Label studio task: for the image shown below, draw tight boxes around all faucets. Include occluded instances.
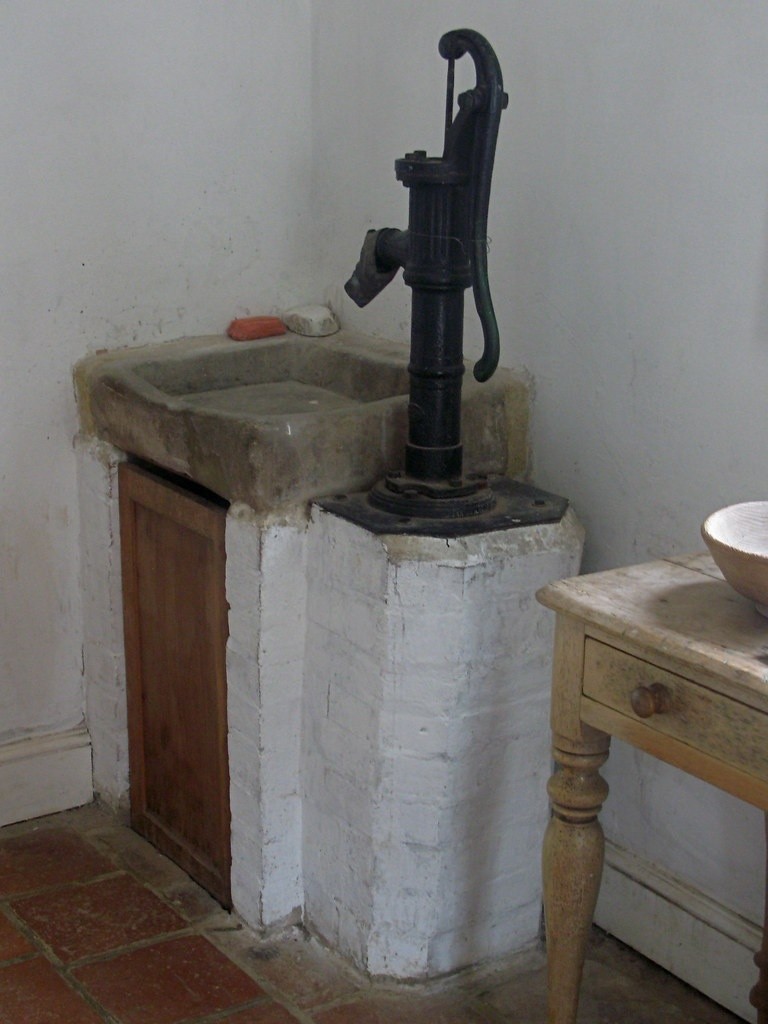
[343,227,404,310]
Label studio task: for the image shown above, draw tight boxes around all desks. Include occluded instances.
[535,550,768,1024]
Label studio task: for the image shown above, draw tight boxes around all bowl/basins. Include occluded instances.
[700,501,768,618]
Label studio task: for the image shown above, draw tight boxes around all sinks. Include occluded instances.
[73,327,532,514]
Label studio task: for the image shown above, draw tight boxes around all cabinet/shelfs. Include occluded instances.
[116,457,232,913]
